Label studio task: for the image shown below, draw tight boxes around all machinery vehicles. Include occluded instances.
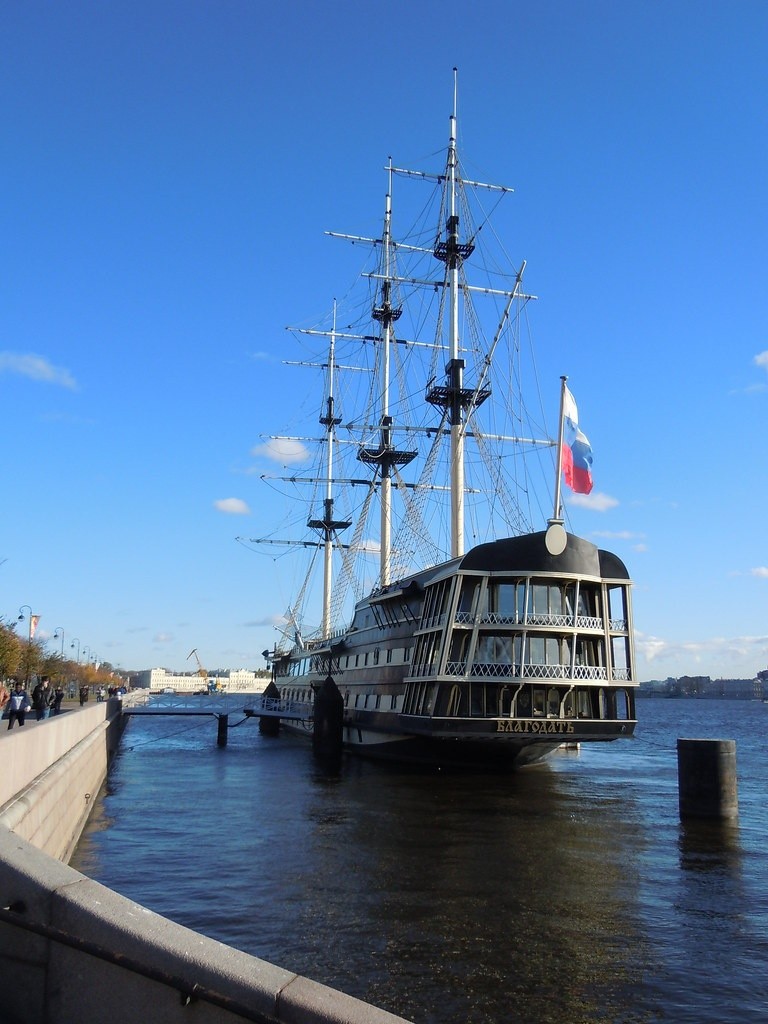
[186,648,227,696]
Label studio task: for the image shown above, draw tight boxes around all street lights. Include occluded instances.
[82,646,105,666]
[53,626,65,661]
[71,637,80,663]
[18,605,33,644]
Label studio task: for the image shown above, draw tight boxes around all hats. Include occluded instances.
[40,676,50,681]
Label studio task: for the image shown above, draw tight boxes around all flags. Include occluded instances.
[559,386,592,494]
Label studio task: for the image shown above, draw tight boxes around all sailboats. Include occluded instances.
[249,67,640,775]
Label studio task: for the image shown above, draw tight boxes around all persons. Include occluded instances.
[0,678,9,722]
[31,676,55,722]
[55,685,128,714]
[6,682,32,731]
